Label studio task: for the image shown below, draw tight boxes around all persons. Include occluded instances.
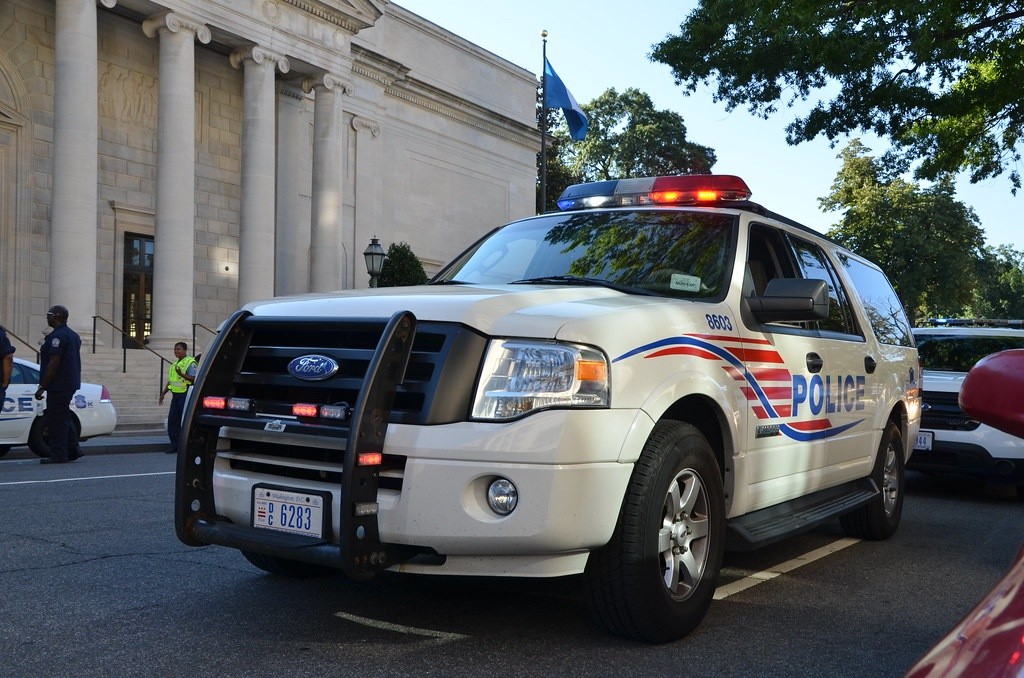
[35,305,85,464]
[159,342,199,453]
[0,325,16,417]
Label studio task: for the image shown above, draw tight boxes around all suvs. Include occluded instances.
[165,174,928,642]
[907,319,1024,486]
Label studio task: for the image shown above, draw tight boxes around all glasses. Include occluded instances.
[47,311,59,317]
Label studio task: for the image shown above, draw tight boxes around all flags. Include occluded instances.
[544,59,588,140]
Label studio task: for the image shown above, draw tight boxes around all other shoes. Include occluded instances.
[41,454,68,464]
[67,447,85,461]
[165,444,177,453]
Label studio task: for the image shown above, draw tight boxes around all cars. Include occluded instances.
[0,354,118,457]
[897,349,1023,678]
